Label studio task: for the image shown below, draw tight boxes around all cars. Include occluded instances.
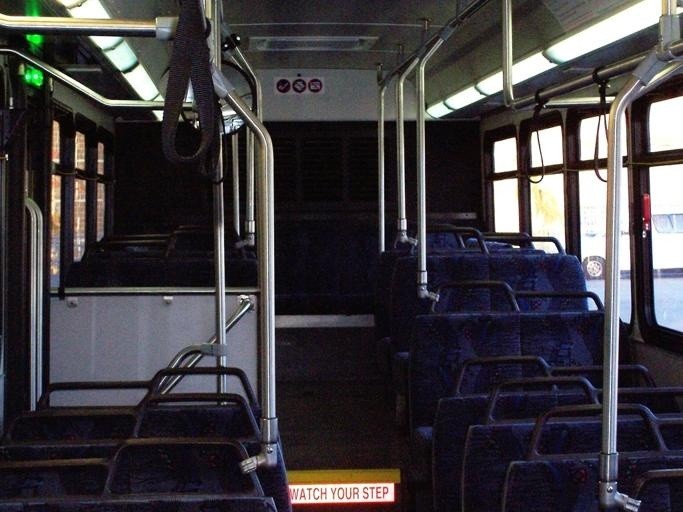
[581,212,683,279]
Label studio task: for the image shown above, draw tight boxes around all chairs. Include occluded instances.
[2,224,683,512]
